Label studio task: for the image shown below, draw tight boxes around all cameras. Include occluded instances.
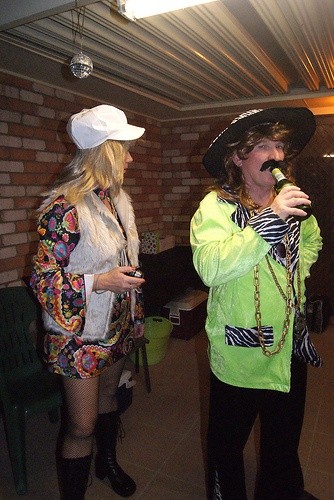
[123,266,145,278]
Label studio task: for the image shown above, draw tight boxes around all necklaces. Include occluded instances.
[243,186,300,357]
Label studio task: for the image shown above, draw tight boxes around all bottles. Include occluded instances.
[272,167,312,221]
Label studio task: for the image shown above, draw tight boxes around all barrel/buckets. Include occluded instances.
[128,317,173,365]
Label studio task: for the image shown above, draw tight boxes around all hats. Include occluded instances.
[66,104,146,150]
[202,105,317,179]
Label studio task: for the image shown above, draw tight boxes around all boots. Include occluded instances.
[56,454,92,500]
[95,410,137,497]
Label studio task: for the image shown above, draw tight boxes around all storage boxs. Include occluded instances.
[129,317,173,365]
[162,286,209,341]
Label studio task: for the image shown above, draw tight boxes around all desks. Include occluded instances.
[136,246,196,308]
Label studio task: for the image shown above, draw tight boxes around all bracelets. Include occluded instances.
[95,273,108,294]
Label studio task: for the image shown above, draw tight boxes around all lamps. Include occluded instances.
[70,0,94,80]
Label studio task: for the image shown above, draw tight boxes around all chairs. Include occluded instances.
[0,286,67,495]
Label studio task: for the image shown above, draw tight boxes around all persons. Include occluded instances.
[190,106,323,500]
[29,105,145,500]
